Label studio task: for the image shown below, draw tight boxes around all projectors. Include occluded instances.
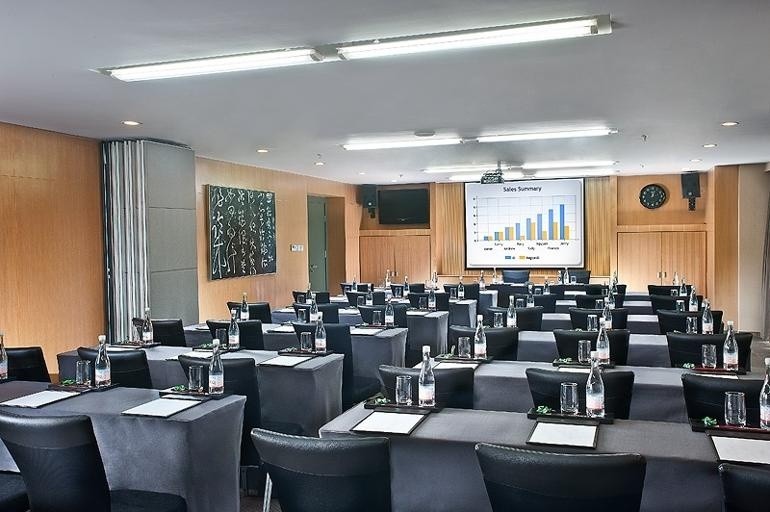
[479,169,505,185]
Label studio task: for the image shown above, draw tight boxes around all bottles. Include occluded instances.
[215,267,499,352]
[597,321,610,365]
[586,271,714,334]
[473,314,487,359]
[492,268,577,329]
[208,339,224,394]
[760,358,770,430]
[419,345,436,405]
[584,351,607,418]
[724,320,739,371]
[94,333,110,387]
[0,333,8,383]
[141,307,154,343]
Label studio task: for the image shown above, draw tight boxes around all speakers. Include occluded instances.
[360,183,377,208]
[680,174,701,199]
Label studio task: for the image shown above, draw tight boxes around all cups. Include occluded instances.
[700,344,717,369]
[395,375,413,405]
[458,335,471,359]
[130,325,141,342]
[187,364,204,391]
[579,340,591,365]
[560,380,579,415]
[75,359,90,384]
[724,390,746,428]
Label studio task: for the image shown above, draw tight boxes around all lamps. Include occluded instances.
[341,118,616,185]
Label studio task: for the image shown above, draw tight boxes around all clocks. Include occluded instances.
[639,182,667,210]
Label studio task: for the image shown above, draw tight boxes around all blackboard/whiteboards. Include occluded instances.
[204,183,276,281]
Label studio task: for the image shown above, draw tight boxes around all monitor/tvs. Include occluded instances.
[378,187,430,225]
